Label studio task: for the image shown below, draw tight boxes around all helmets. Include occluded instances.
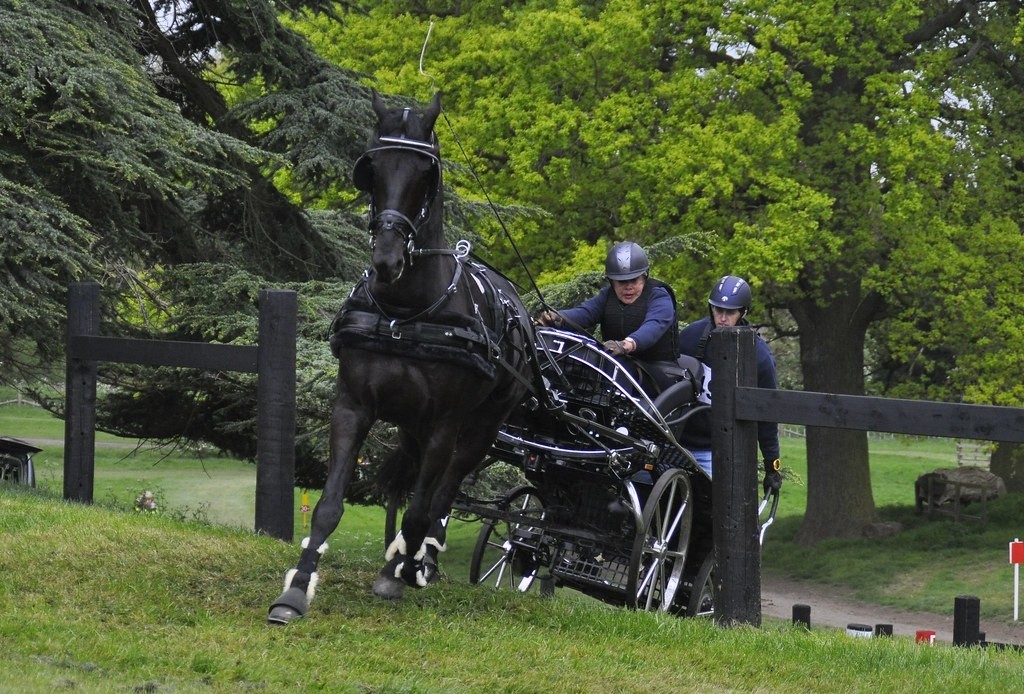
[605,242,649,281]
[708,274,751,309]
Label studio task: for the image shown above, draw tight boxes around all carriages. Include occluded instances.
[267,87,781,624]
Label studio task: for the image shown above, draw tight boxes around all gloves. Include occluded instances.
[763,458,782,497]
[603,339,627,357]
[539,308,563,329]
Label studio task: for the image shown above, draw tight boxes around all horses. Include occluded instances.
[269,87,535,627]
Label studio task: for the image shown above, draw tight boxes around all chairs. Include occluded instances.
[634,351,704,441]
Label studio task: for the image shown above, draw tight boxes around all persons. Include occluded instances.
[679,276,784,494]
[533,240,682,577]
